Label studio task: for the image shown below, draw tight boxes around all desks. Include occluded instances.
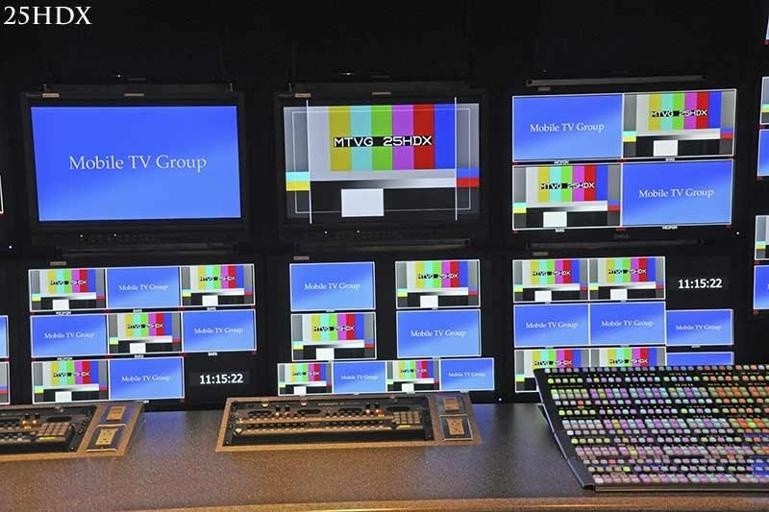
[0,402,769,512]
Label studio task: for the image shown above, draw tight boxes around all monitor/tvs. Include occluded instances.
[0,77,769,412]
